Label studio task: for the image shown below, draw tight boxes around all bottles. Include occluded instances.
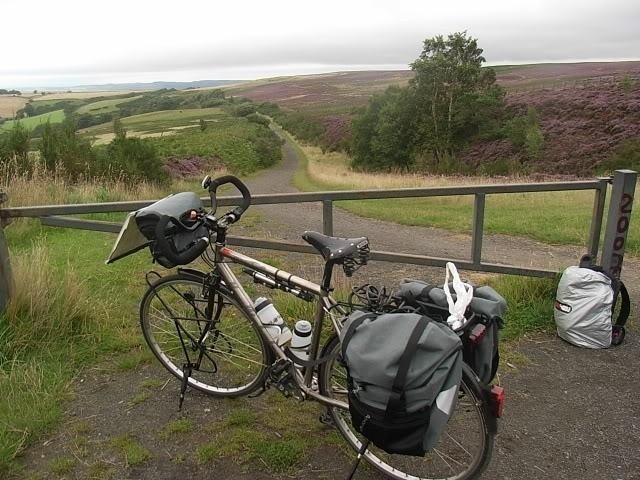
[252,295,313,370]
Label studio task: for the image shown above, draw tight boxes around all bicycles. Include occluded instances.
[140,174,508,480]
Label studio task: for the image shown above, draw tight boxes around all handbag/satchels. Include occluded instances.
[554,254,630,350]
[338,310,465,457]
[398,277,507,383]
